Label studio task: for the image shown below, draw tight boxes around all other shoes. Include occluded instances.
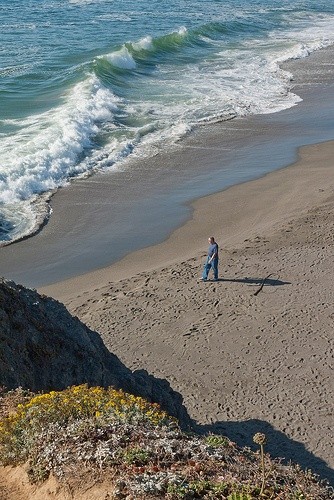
[199,277,207,282]
[211,277,218,281]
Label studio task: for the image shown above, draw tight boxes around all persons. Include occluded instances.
[199,237,219,282]
